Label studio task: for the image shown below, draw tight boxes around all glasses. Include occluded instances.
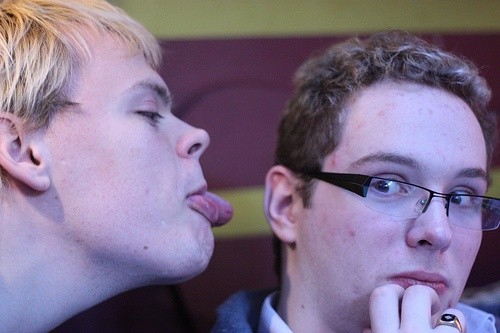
[299,159,500,231]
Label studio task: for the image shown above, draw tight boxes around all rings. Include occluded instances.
[434,313,463,333]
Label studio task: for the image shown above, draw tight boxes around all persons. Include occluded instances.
[209,27,500,333]
[0,0,234,333]
